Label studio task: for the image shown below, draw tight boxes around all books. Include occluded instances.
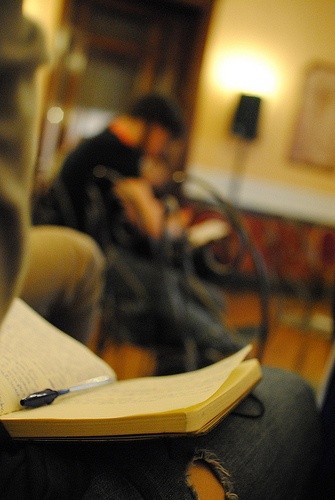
[1,295,263,442]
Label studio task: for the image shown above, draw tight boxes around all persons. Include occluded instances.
[1,365,319,500]
[39,95,244,363]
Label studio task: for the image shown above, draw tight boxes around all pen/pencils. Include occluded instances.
[19,376,113,408]
